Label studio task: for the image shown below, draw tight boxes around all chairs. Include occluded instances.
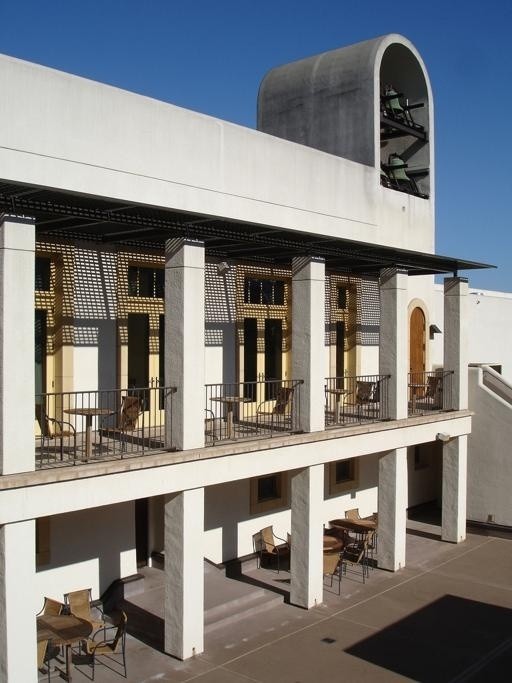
[342,380,376,424]
[34,403,77,461]
[256,507,377,596]
[85,611,129,681]
[63,588,108,642]
[36,596,66,618]
[254,387,295,437]
[204,408,218,442]
[425,376,443,411]
[97,395,146,457]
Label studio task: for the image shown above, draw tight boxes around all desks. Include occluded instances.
[408,384,424,413]
[37,615,93,683]
[210,397,253,439]
[61,408,115,457]
[325,389,351,423]
